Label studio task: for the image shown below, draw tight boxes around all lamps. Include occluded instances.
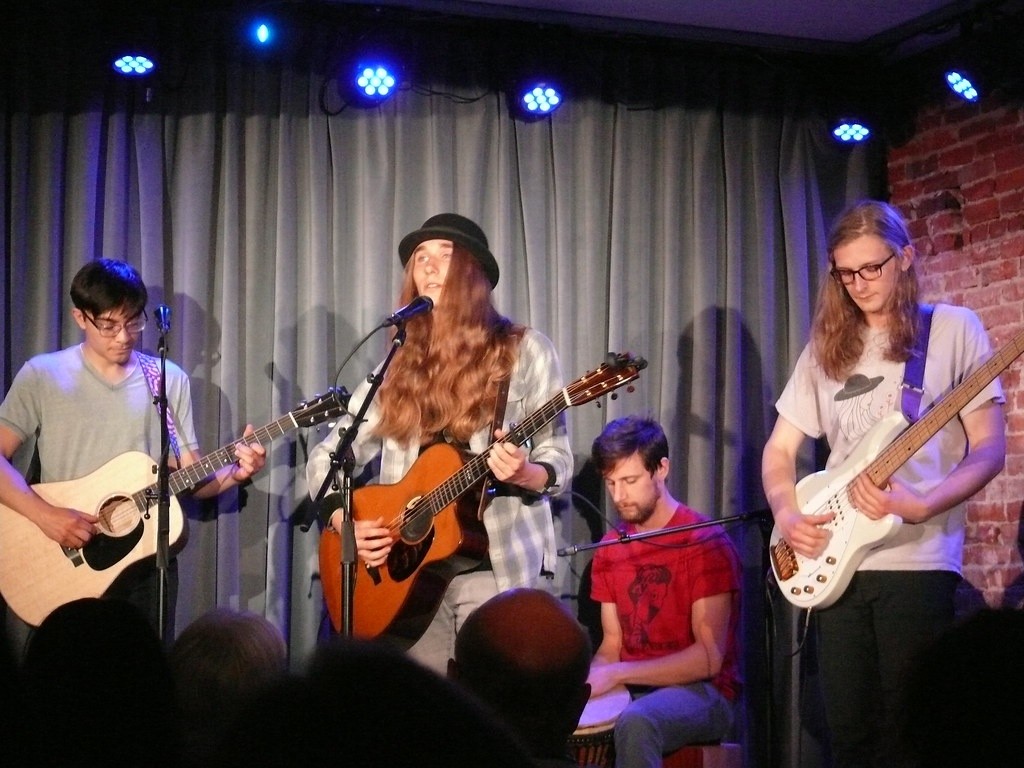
[338,27,406,110]
[942,26,1016,103]
[108,4,162,82]
[826,69,879,144]
[491,38,575,123]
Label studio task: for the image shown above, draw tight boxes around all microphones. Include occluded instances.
[382,296,433,327]
[154,304,170,334]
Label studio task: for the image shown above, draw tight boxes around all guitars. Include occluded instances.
[0,382,351,635]
[766,325,1023,613]
[314,347,650,653]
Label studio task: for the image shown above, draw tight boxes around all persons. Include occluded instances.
[305,214,572,672]
[760,203,1004,768]
[577,417,742,768]
[1,588,593,768]
[0,258,269,636]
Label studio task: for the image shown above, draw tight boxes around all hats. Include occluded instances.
[398,213,500,290]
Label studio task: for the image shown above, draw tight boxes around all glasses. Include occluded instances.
[83,309,149,338]
[831,254,894,285]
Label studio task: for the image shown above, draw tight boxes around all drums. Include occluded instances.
[563,666,633,767]
